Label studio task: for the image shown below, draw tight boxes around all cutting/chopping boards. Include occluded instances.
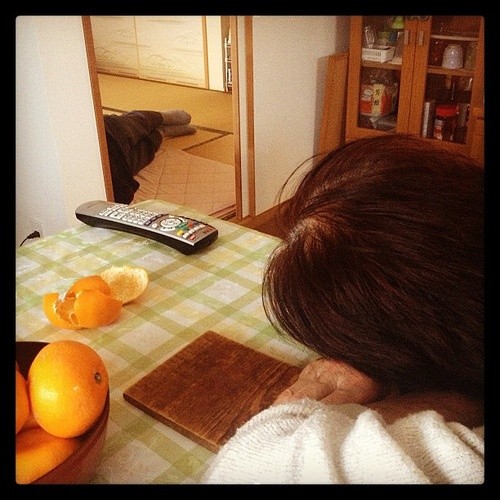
[123,329,302,453]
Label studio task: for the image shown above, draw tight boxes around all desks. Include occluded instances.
[15,198,325,484]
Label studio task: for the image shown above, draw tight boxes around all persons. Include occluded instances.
[104,111,197,205]
[263,142,485,428]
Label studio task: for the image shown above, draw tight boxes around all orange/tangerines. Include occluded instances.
[15,339,109,485]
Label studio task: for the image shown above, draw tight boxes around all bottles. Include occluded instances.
[428,40,442,66]
[433,75,474,144]
[359,68,399,117]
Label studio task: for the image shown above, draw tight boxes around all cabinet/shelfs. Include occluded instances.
[346,17,484,163]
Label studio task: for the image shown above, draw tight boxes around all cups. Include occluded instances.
[377,28,396,46]
[442,43,463,70]
[464,41,477,71]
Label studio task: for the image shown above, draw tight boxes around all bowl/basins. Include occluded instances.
[16,340,110,484]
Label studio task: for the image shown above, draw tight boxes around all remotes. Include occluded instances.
[75,200,218,254]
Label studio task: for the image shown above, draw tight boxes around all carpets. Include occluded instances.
[129,147,237,214]
